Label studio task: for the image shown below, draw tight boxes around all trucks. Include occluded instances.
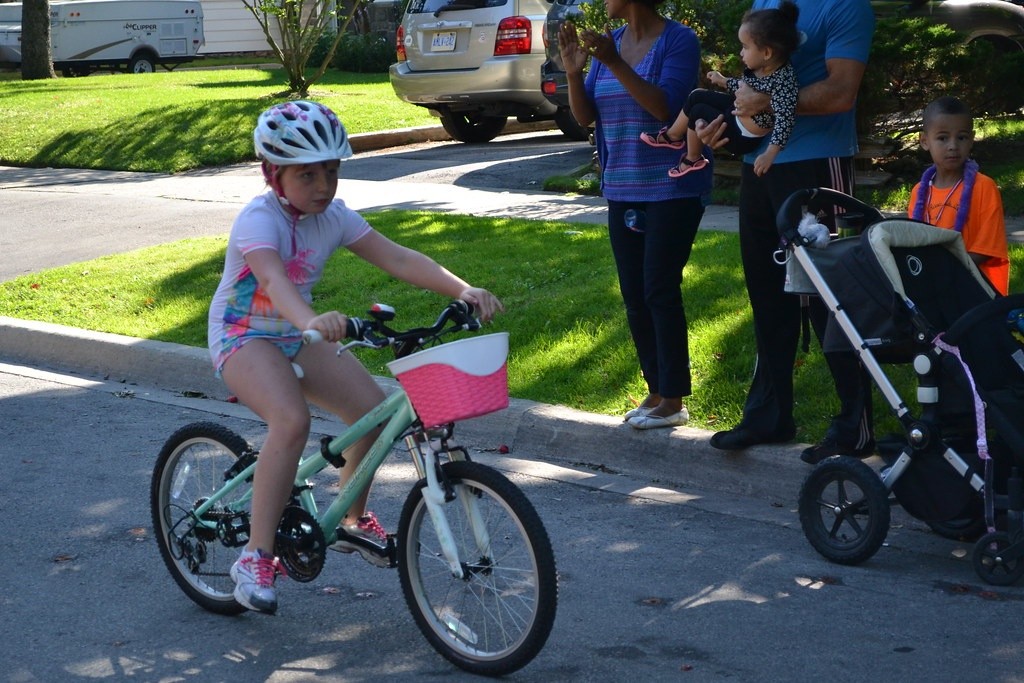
[2,0,205,76]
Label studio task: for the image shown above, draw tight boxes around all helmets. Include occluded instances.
[255,101,354,164]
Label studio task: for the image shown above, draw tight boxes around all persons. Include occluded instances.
[905,95,1011,303]
[694,0,878,464]
[207,99,505,615]
[557,0,715,430]
[638,7,800,178]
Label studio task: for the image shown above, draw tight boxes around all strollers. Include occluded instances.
[772,188,1023,586]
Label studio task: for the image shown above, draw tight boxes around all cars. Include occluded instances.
[390,0,591,141]
[540,0,1023,111]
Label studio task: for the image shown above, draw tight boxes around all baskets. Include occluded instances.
[388,331,512,428]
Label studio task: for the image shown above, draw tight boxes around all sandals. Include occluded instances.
[669,154,710,177]
[639,132,686,149]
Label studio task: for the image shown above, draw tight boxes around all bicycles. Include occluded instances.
[151,299,560,677]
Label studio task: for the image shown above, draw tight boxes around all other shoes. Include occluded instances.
[625,406,690,427]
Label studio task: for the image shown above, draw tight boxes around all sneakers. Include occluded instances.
[331,514,399,568]
[799,432,879,462]
[710,426,798,449]
[231,543,279,613]
